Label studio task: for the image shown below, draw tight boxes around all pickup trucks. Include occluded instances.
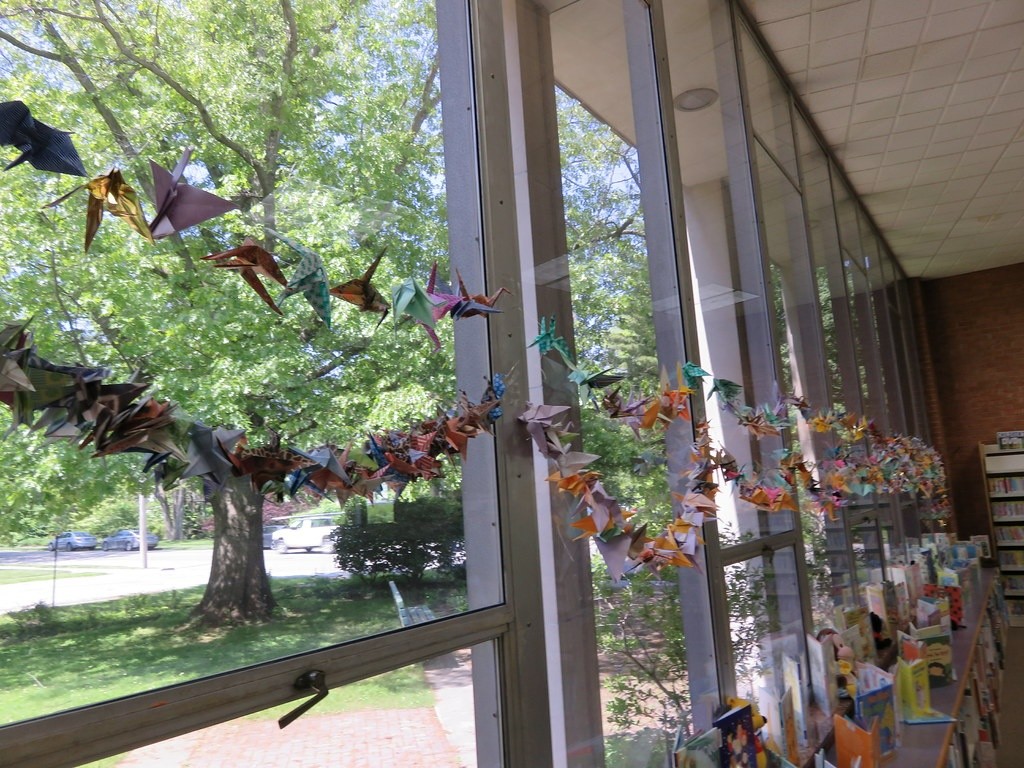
[271,517,341,554]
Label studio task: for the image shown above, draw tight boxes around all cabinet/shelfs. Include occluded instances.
[806,442,1024,768]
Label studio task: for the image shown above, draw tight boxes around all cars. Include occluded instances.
[48,531,98,551]
[102,528,159,552]
[263,516,294,550]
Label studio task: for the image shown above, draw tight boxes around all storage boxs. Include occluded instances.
[997,430,1024,451]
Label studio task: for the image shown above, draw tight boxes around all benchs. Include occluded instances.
[389,580,437,628]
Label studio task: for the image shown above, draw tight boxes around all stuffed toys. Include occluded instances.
[816,628,858,698]
[727,698,768,768]
[869,611,893,650]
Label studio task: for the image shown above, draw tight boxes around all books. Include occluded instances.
[815,749,825,768]
[989,476,1024,617]
[855,534,1008,768]
[833,715,881,768]
[671,704,758,768]
[759,687,799,765]
[806,634,839,716]
[782,652,820,748]
[824,755,862,768]
[997,431,1024,450]
